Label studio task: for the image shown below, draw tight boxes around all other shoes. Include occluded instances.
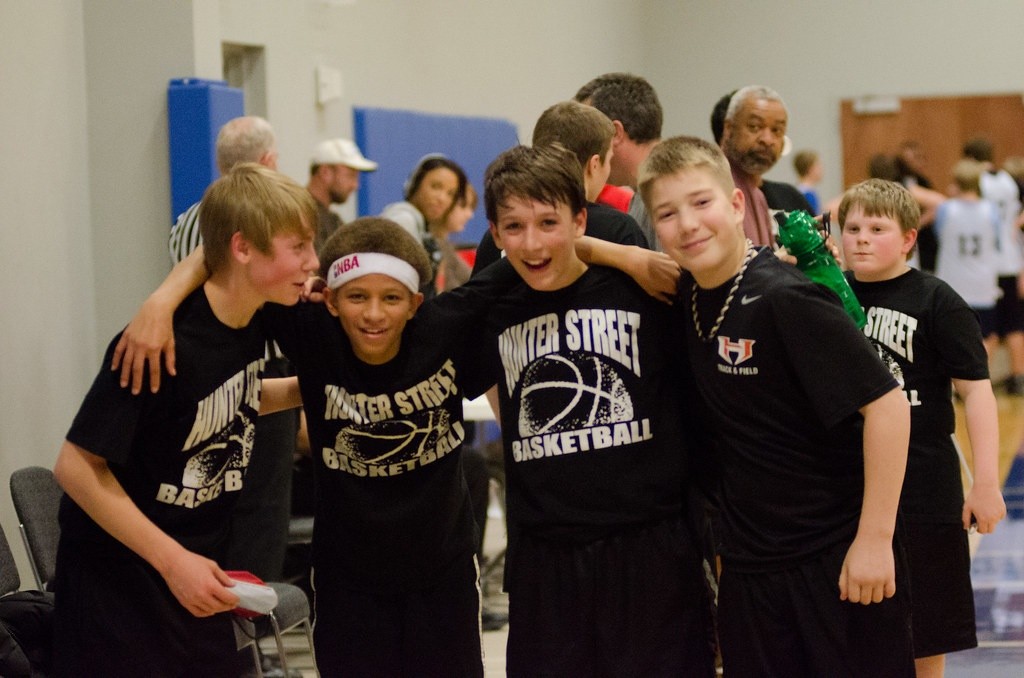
[1006,372,1023,397]
[481,604,509,631]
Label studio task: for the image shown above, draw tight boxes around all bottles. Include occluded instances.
[773,209,867,328]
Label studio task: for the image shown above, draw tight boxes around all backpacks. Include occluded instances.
[0,590,56,678]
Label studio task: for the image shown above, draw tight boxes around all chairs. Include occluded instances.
[0,462,315,678]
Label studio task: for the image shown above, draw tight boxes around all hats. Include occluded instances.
[310,138,379,172]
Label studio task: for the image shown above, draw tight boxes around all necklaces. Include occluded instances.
[692,239,752,345]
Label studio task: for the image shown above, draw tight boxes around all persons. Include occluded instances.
[111,218,681,678]
[52,161,320,678]
[638,135,916,678]
[839,178,1005,678]
[169,76,1024,633]
[299,146,717,678]
